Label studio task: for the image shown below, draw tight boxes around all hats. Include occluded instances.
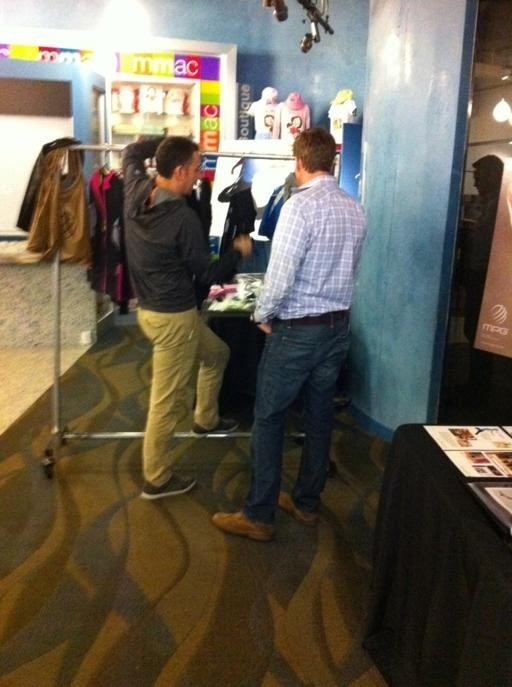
[335,89,353,103]
[287,92,303,109]
[262,87,277,99]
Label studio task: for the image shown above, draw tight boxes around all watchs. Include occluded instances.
[249,314,262,326]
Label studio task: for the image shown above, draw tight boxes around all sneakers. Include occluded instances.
[141,472,197,498]
[192,418,240,436]
[278,491,319,525]
[211,510,271,541]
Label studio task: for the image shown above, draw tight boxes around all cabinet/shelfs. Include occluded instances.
[104,73,202,173]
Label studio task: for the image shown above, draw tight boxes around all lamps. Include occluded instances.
[261,0,321,54]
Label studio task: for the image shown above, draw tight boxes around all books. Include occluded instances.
[466,481,511,531]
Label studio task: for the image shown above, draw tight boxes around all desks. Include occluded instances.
[353,411,512,687]
[202,287,268,435]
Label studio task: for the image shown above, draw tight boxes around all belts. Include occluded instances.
[290,311,343,324]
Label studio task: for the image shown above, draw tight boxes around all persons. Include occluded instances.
[210,127,366,542]
[286,116,302,138]
[458,154,503,421]
[263,115,274,132]
[121,135,253,501]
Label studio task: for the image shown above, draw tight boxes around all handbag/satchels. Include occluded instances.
[16,137,93,264]
